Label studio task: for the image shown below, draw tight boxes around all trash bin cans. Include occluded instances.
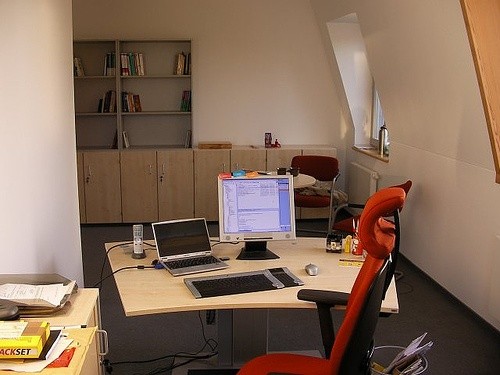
[367,345,429,375]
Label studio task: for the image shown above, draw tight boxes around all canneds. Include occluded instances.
[265,133,272,148]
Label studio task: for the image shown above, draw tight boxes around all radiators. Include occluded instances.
[348,161,379,204]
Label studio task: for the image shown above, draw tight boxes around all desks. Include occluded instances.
[80,327,108,375]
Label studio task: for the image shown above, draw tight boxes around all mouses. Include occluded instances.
[305,263,319,276]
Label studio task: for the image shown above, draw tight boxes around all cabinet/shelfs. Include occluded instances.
[121,150,194,224]
[78,151,120,224]
[74,37,120,148]
[87,295,101,326]
[195,150,267,222]
[119,37,196,149]
[104,236,400,374]
[267,149,336,220]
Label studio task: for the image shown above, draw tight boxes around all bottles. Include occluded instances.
[379,122,387,154]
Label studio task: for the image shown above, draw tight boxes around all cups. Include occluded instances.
[290,166,300,177]
[276,167,286,175]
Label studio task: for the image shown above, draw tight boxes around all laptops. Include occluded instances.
[151,218,229,277]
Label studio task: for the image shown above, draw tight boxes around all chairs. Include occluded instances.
[289,155,341,237]
[235,180,412,375]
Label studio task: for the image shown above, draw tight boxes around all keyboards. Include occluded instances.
[184,267,305,299]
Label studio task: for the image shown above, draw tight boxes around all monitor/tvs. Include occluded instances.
[218,175,296,260]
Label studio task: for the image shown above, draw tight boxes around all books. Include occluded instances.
[185,129,191,148]
[96,91,116,112]
[122,131,130,148]
[111,129,116,148]
[174,52,190,75]
[120,53,145,75]
[74,56,85,76]
[39,330,62,360]
[122,92,142,112]
[180,91,190,112]
[103,52,115,76]
[197,143,232,149]
